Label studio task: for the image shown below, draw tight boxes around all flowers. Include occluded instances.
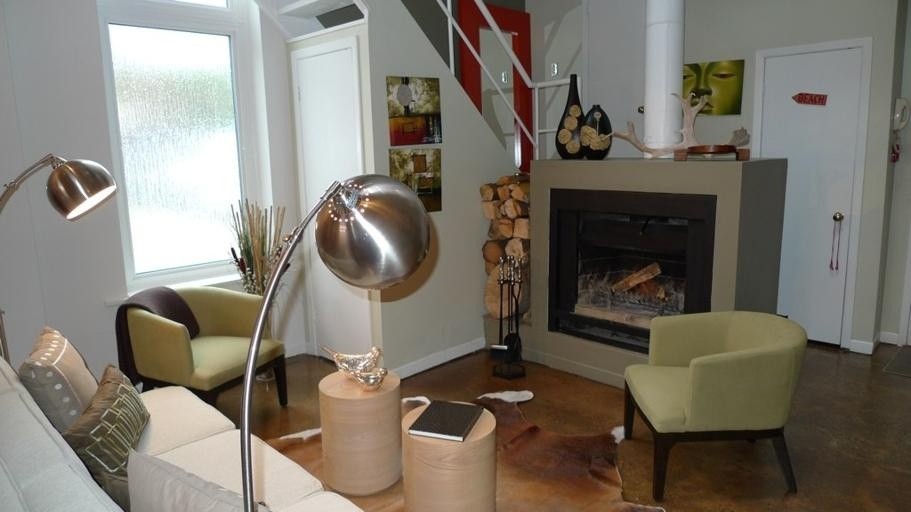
[226,197,291,382]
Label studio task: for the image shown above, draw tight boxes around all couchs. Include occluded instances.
[1,355,364,511]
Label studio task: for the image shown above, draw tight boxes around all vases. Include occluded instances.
[555,72,613,161]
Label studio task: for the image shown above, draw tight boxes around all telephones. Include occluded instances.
[893,98,910,130]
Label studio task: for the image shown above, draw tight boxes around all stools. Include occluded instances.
[318,368,496,511]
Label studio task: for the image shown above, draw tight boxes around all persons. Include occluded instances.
[684,61,743,116]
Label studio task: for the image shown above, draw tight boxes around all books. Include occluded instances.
[408,400,483,442]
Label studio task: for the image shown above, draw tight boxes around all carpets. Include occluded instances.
[264,389,665,510]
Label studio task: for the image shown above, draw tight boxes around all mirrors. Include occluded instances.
[476,24,524,169]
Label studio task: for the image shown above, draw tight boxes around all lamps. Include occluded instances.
[1,152,119,222]
[236,172,437,512]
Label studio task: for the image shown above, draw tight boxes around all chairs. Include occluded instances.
[623,309,809,502]
[125,284,287,407]
